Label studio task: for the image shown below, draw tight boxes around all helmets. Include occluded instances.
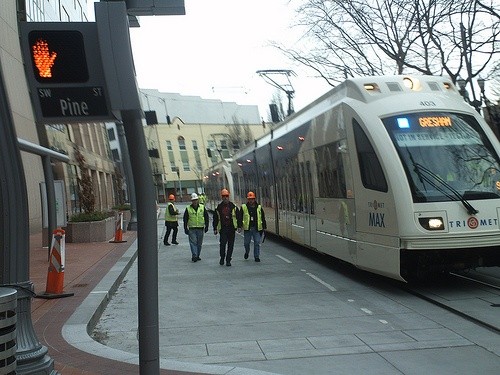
[247,192,255,198]
[190,192,198,200]
[221,189,229,195]
[169,195,175,199]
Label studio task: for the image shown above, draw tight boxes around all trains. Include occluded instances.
[201,75,500,284]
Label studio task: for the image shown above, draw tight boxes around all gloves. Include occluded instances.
[204,227,208,233]
[214,230,218,235]
[237,228,241,233]
[185,229,189,234]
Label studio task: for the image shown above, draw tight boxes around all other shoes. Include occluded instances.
[172,241,179,244]
[226,260,231,266]
[192,257,197,262]
[244,252,249,259]
[164,242,171,246]
[220,257,225,265]
[255,258,260,261]
[197,255,202,260]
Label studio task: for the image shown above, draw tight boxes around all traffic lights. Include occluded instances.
[18,21,110,124]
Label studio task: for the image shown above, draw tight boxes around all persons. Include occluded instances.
[213,188,243,266]
[164,195,179,246]
[239,191,266,262]
[183,193,209,261]
[430,154,459,192]
